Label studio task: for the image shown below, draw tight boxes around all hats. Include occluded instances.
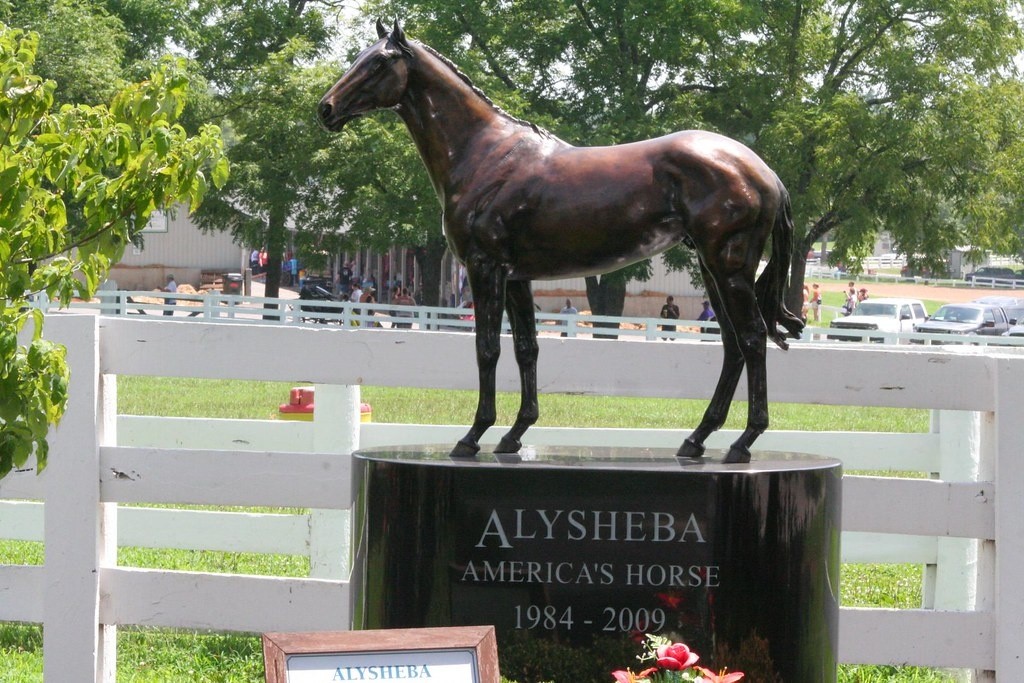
[701,301,709,307]
[363,286,376,292]
[859,288,867,294]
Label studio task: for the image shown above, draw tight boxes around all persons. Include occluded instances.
[560,298,577,337]
[342,283,377,327]
[697,301,715,334]
[156,274,177,315]
[660,296,679,341]
[801,283,822,322]
[842,282,857,315]
[251,246,306,290]
[389,285,417,328]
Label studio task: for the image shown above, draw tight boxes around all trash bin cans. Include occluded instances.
[221,272,243,306]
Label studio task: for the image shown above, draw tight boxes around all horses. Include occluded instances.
[315,15,809,466]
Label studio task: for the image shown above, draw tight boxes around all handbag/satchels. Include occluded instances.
[841,306,848,315]
[817,300,821,304]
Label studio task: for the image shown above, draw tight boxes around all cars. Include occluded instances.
[964,265,1014,286]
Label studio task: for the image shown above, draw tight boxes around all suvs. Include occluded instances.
[908,297,1024,348]
[822,298,927,345]
